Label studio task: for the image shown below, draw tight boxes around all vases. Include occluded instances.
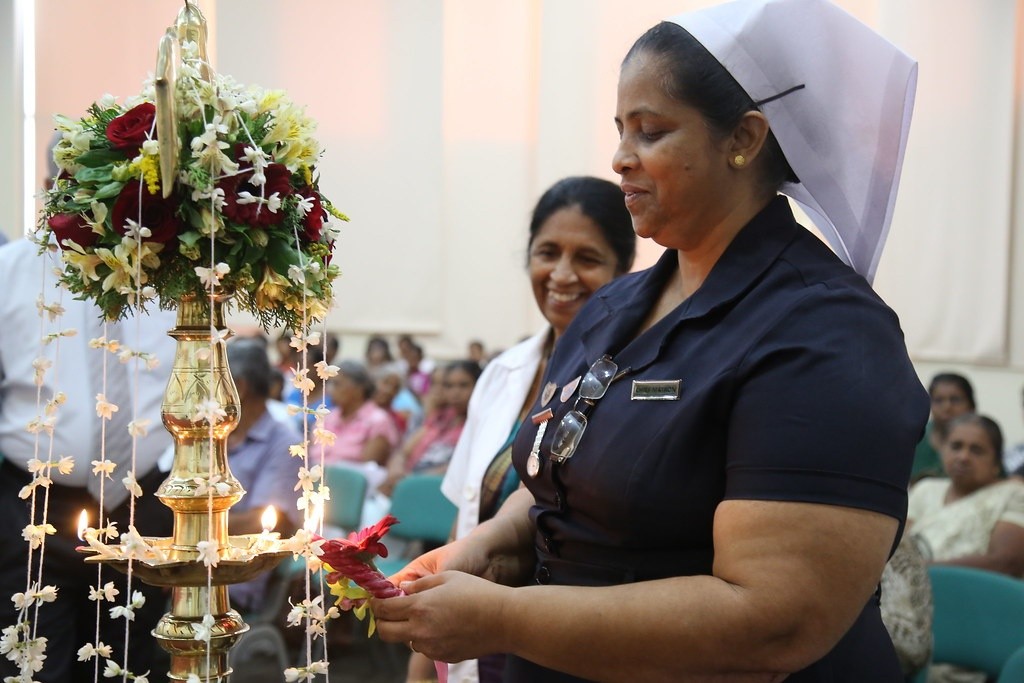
[71,289,301,683]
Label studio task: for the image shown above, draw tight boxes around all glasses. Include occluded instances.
[548,352,619,462]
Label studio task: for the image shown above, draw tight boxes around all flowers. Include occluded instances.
[29,39,350,341]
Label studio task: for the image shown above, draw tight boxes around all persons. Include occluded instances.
[0,133,181,682]
[368,0,932,683]
[440,176,636,678]
[221,335,1023,625]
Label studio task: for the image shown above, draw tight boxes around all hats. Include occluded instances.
[217,336,285,387]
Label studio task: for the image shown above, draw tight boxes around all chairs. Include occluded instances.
[915,562,1023,683]
[260,465,367,624]
[298,473,460,670]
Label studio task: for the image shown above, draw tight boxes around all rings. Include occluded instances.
[409,641,419,653]
